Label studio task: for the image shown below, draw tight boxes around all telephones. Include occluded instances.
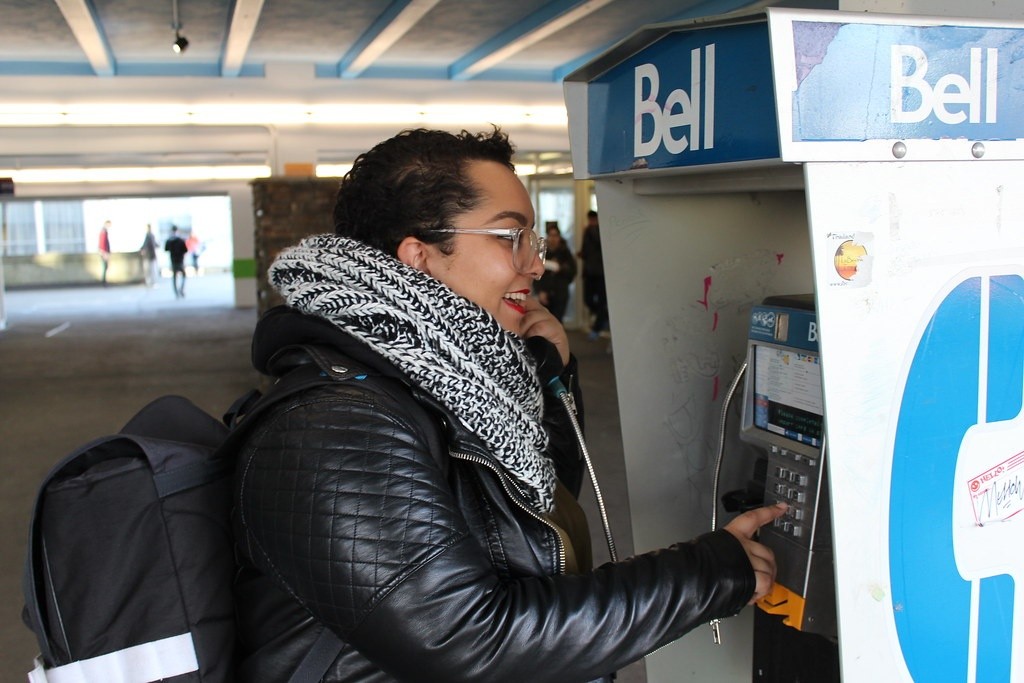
[522,329,568,406]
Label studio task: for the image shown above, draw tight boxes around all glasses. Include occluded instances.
[419,227,547,280]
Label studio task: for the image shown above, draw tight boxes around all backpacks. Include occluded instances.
[17,377,445,683]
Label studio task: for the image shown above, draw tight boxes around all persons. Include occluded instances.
[218,128,788,683]
[138,222,202,299]
[575,209,609,339]
[535,221,578,321]
[96,219,114,285]
